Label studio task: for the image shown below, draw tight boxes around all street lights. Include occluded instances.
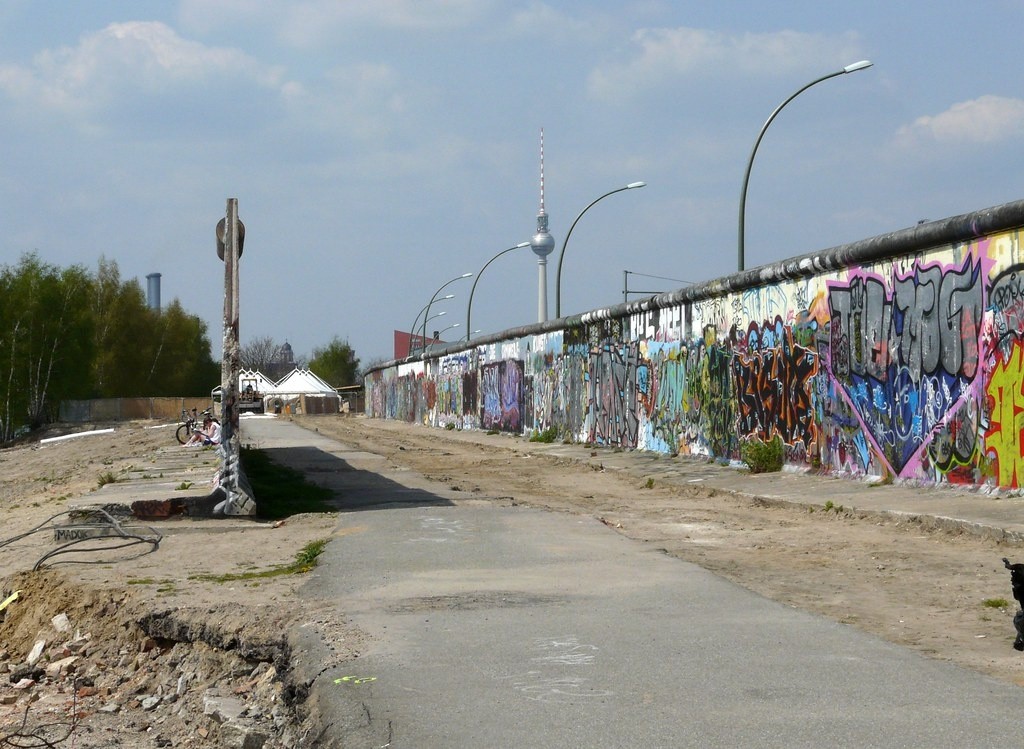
[405,272,482,355]
[466,241,533,343]
[736,59,875,271]
[554,179,648,318]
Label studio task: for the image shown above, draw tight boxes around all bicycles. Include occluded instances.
[175,407,200,445]
[199,405,215,424]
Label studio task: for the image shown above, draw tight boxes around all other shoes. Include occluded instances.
[180,445,189,447]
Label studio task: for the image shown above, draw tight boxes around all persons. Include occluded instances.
[179,416,222,448]
[192,418,220,447]
